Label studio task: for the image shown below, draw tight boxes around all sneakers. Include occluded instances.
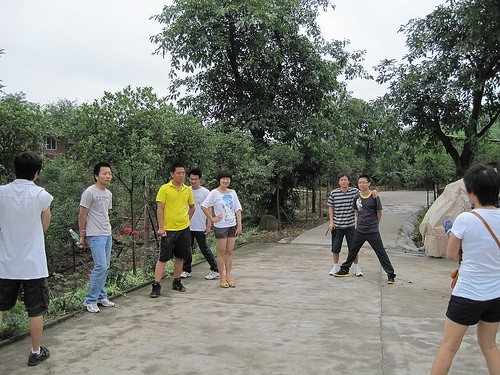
[27,347,49,366]
[353,267,363,276]
[333,269,350,276]
[387,276,394,284]
[180,271,190,278]
[329,266,340,275]
[150,283,161,298]
[205,271,220,279]
[172,278,186,291]
[96,297,115,307]
[83,300,100,313]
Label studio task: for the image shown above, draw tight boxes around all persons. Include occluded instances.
[327,172,378,276]
[0,152,54,365]
[77,162,115,312]
[150,164,196,297]
[431,162,500,375]
[334,174,396,283]
[180,170,220,280]
[201,172,242,287]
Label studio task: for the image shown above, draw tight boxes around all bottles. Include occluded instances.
[69,229,80,243]
[443,219,454,238]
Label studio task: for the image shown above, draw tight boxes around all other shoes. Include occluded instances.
[226,279,235,287]
[219,279,229,287]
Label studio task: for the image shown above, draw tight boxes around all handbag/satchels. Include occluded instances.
[450,268,459,288]
